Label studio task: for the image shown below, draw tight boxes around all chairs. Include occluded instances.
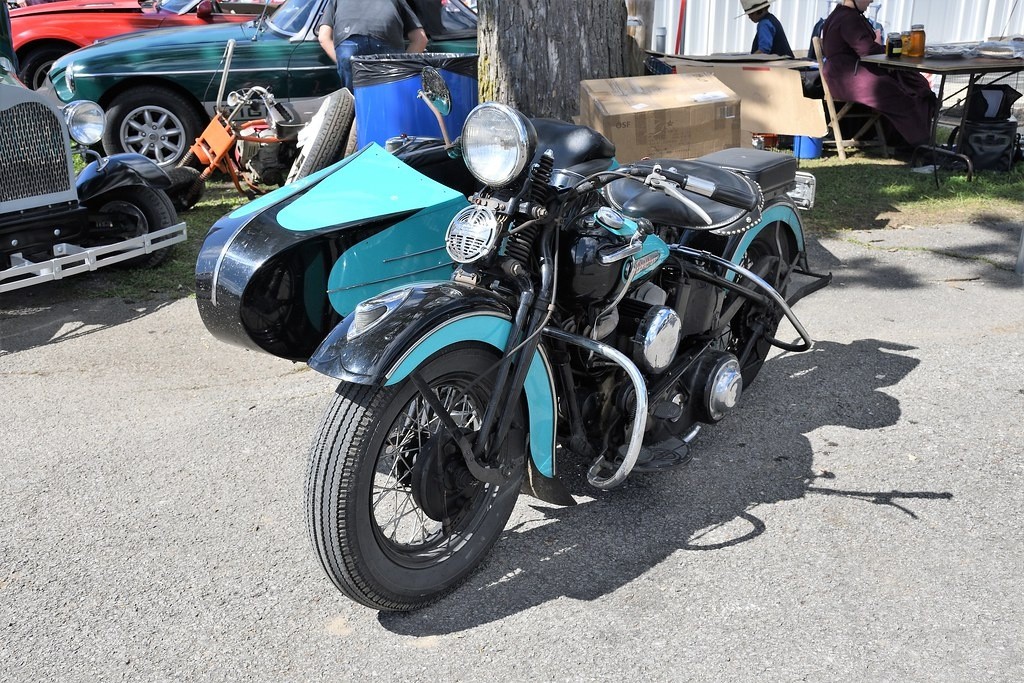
[812,36,888,163]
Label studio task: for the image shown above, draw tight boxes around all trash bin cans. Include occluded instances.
[348,52,480,157]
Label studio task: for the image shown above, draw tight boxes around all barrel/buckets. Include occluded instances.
[793,135,823,159]
[349,53,480,153]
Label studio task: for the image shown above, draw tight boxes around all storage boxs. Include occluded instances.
[568,71,742,166]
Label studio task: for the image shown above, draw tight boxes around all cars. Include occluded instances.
[8,0,290,92]
[45,0,482,174]
[1,0,190,303]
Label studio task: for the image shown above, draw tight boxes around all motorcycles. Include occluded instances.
[160,37,311,212]
[195,76,843,618]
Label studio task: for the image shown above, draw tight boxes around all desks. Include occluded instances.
[861,41,1024,183]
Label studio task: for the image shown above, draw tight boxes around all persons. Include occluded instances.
[821,0,938,152]
[734,0,795,59]
[317,0,428,95]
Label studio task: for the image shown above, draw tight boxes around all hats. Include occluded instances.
[734,1,776,20]
